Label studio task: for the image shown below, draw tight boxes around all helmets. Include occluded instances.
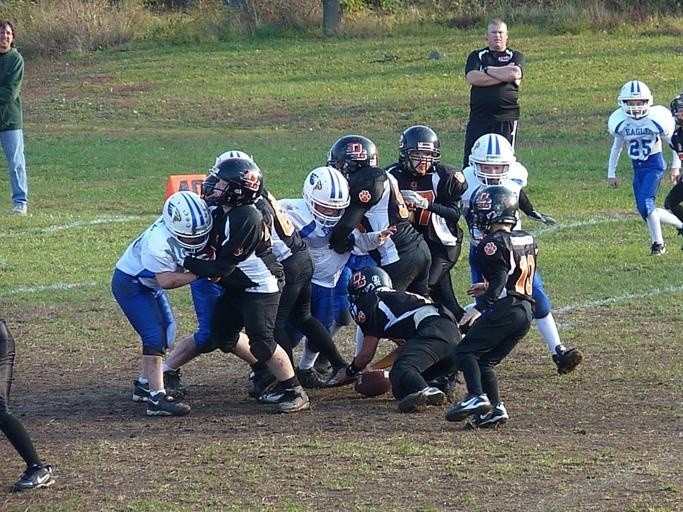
[467,134,516,187]
[327,133,381,180]
[202,151,262,207]
[301,165,351,228]
[396,123,443,176]
[470,182,522,236]
[670,94,683,127]
[162,191,212,254]
[618,81,654,120]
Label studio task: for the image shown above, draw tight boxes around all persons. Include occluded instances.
[665,93,683,238]
[113,125,582,428]
[0,321,55,489]
[606,79,683,256]
[0,21,28,214]
[464,16,523,168]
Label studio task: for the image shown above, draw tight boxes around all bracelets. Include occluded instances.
[483,66,488,73]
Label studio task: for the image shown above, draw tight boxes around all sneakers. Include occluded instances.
[395,372,458,413]
[248,359,355,415]
[12,205,28,217]
[145,393,193,418]
[10,463,55,493]
[447,392,509,431]
[551,344,581,376]
[650,242,669,258]
[133,366,187,402]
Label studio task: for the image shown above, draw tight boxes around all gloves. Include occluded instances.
[527,209,557,226]
[400,188,430,211]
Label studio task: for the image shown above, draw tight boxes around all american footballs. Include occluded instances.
[355,370,390,396]
